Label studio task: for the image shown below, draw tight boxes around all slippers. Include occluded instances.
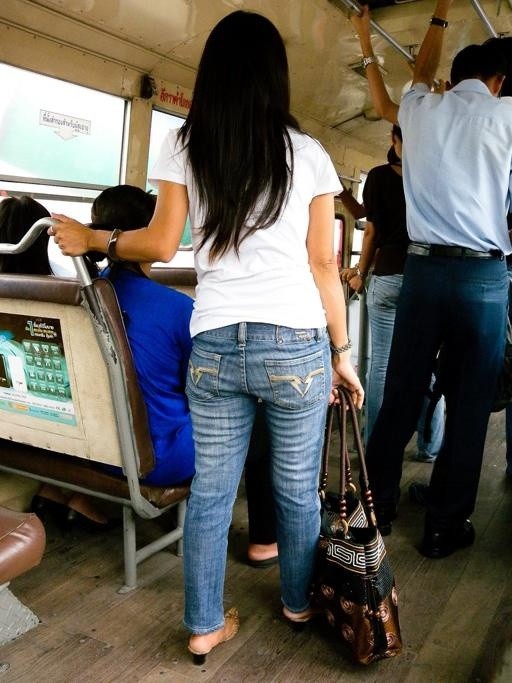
[241,545,280,570]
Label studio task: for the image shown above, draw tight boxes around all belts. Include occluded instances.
[406,242,506,262]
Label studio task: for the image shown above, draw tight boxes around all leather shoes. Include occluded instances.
[372,502,400,537]
[418,519,476,559]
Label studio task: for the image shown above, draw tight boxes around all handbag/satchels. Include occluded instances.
[316,384,404,666]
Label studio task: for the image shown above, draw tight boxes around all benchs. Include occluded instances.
[0,217,191,594]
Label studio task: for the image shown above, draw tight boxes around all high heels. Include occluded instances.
[30,495,124,539]
[187,607,240,666]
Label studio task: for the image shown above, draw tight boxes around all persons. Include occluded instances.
[341,0,512,558]
[86,184,279,569]
[46,10,364,666]
[0,198,116,529]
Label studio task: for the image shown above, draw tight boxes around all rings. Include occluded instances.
[50,226,55,236]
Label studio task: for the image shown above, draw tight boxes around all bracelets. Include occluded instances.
[107,228,126,263]
[330,337,352,353]
